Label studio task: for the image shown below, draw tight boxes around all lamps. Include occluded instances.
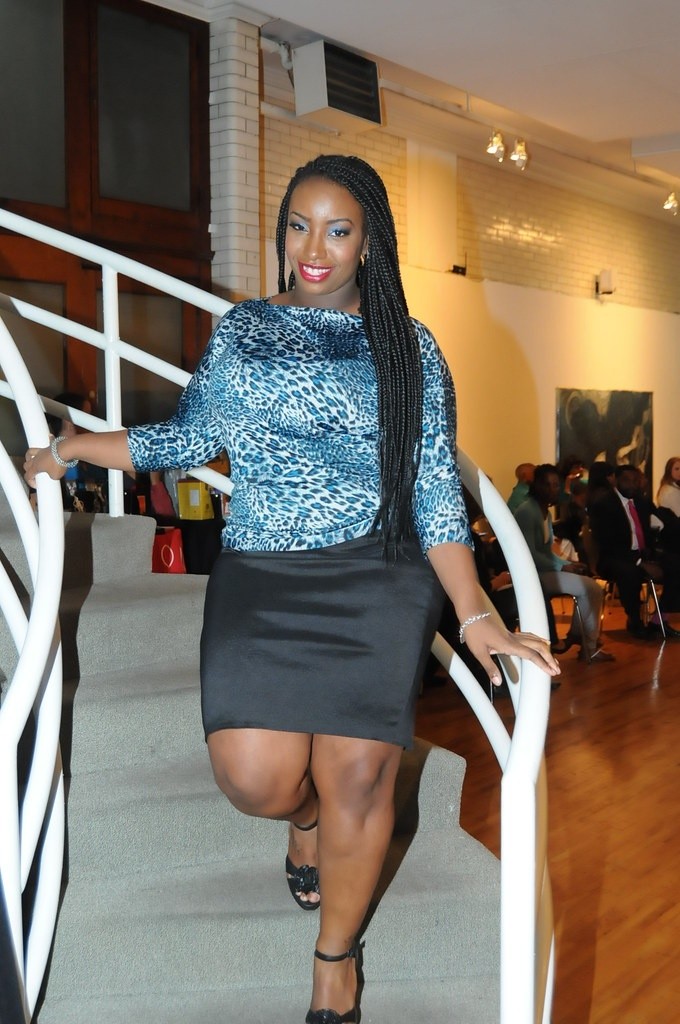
[664,192,679,215]
[511,139,527,171]
[486,134,505,162]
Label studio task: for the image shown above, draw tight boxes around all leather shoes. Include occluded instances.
[660,622,680,638]
[637,629,647,637]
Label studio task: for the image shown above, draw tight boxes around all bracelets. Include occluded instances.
[52,435,82,468]
[459,613,494,642]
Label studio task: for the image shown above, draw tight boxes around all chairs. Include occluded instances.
[491,526,667,706]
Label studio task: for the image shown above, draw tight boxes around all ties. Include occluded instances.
[628,501,646,549]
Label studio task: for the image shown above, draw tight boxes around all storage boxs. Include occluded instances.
[177,479,214,520]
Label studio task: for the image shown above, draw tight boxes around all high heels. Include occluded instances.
[552,639,572,654]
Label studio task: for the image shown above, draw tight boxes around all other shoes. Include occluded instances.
[552,680,561,690]
[591,649,614,661]
[494,685,509,698]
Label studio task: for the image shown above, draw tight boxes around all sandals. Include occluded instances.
[305,938,357,1024]
[286,818,321,911]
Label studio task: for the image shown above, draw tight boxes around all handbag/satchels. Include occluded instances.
[73,491,104,513]
[640,583,663,627]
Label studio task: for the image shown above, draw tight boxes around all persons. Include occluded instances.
[24,153,565,1024]
[503,455,679,660]
